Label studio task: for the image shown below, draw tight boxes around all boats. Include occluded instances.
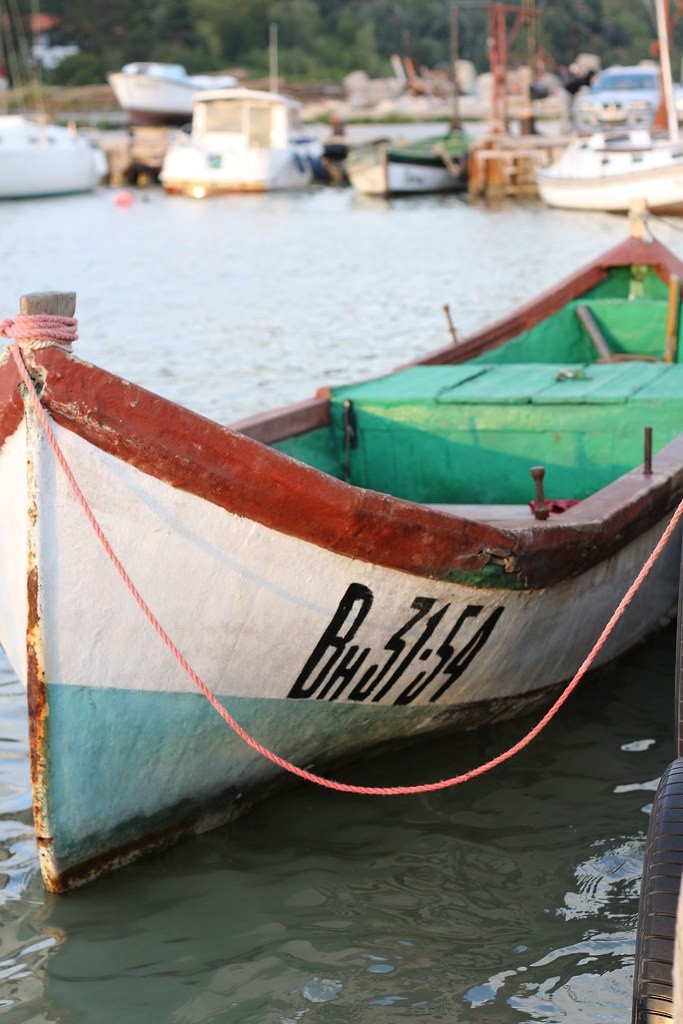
[0,193,683,893]
[0,1,683,217]
